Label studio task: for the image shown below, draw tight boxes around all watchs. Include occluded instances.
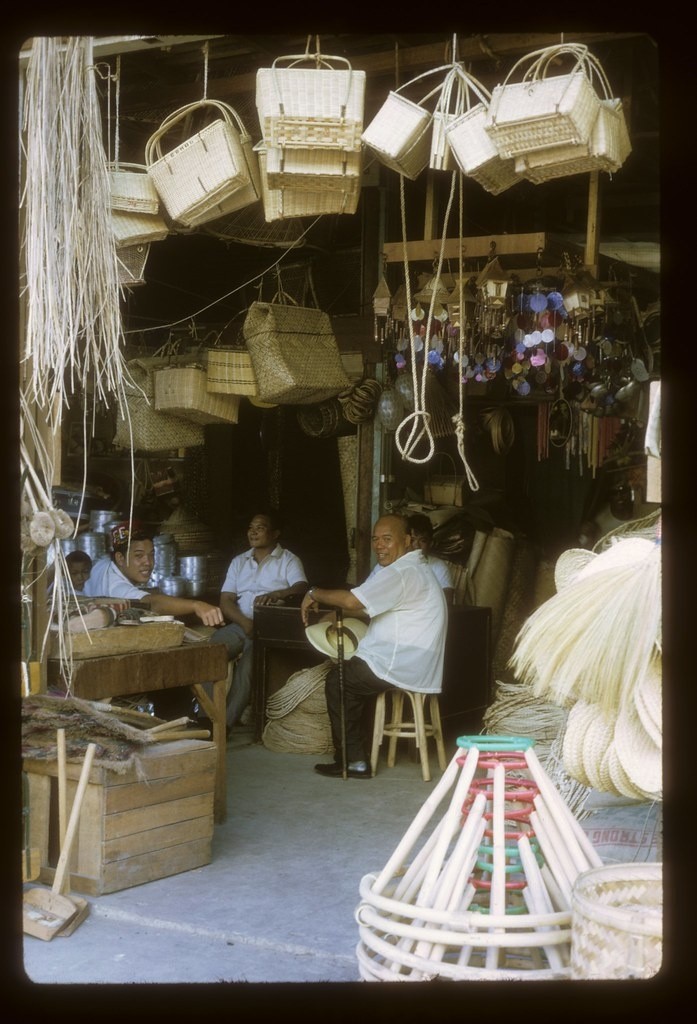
[308,585,319,602]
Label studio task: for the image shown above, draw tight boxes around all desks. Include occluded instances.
[45,641,230,825]
[252,603,491,764]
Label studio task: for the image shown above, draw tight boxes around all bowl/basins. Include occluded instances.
[60,509,122,566]
[142,534,209,597]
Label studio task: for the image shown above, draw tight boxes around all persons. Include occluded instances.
[192,511,308,739]
[301,516,450,779]
[47,551,92,597]
[364,515,454,608]
[82,520,231,736]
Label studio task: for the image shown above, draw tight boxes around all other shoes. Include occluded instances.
[186,717,214,742]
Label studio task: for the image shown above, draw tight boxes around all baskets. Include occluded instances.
[423,452,468,507]
[102,43,633,285]
[111,291,351,452]
[148,467,176,497]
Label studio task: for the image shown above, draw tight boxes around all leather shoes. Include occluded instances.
[314,758,371,779]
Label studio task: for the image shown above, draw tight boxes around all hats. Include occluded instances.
[160,506,213,553]
[305,611,370,667]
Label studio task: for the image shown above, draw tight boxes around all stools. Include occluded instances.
[369,687,447,782]
